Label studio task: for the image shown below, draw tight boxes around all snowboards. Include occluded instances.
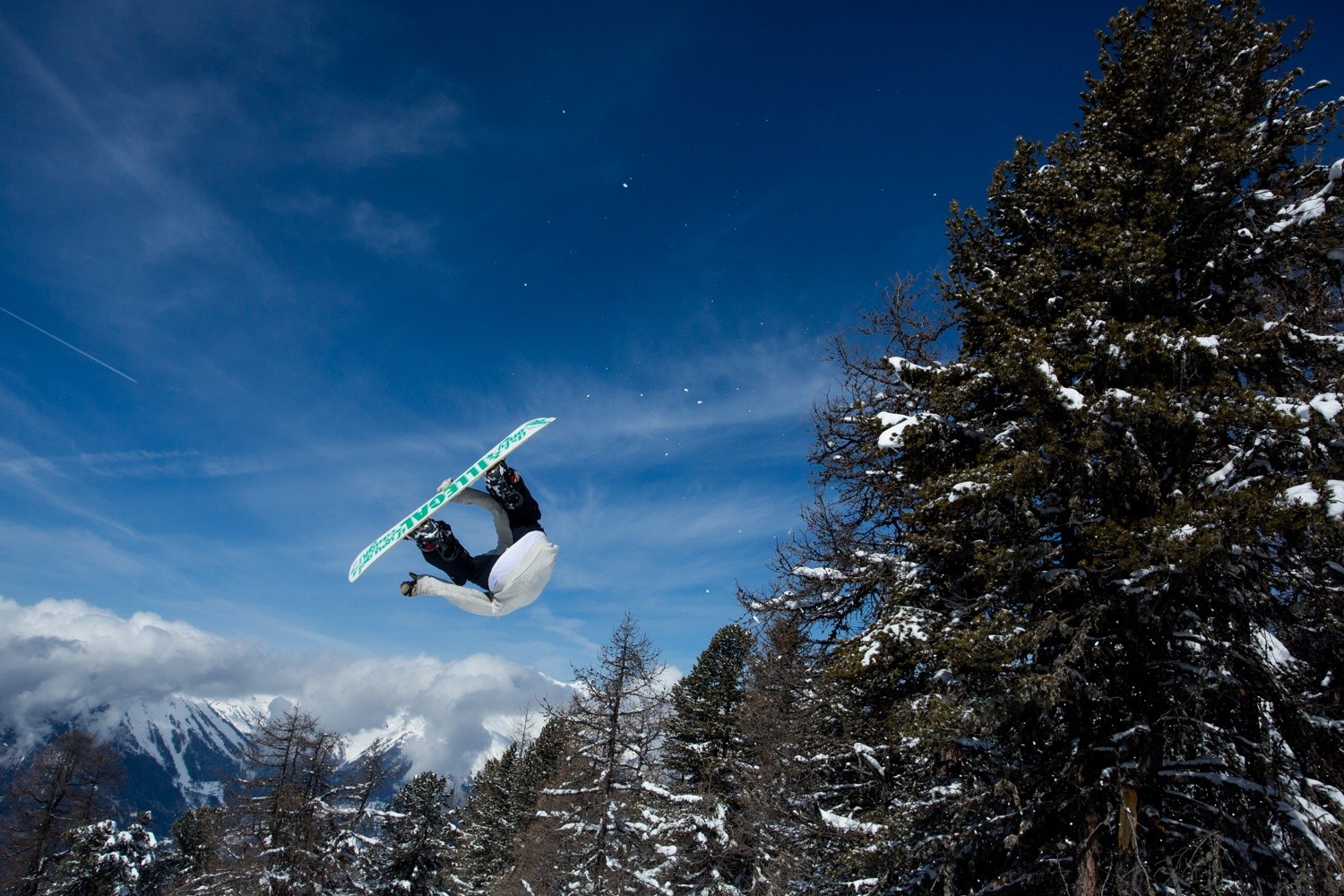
[348,416,557,582]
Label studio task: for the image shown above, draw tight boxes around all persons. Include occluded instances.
[400,456,562,617]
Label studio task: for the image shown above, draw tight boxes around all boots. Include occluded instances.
[412,521,471,586]
[491,461,539,527]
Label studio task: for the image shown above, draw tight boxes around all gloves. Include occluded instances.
[435,477,453,492]
[400,572,417,597]
